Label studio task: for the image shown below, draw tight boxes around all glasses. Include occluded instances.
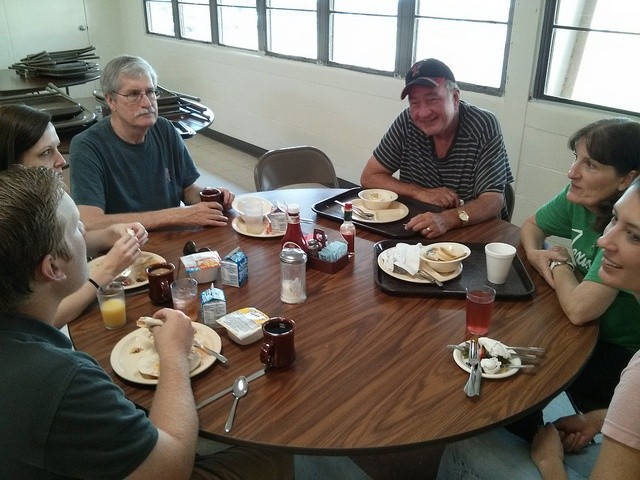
[183,240,210,254]
[110,89,157,103]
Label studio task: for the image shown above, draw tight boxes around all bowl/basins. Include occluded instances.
[358,189,399,210]
[231,195,275,223]
[420,240,471,274]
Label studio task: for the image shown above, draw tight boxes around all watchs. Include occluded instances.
[454,208,470,227]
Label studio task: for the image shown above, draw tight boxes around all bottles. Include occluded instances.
[279,204,310,254]
[308,239,318,257]
[279,248,308,305]
[339,203,356,258]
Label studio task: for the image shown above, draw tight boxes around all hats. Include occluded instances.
[401,58,455,100]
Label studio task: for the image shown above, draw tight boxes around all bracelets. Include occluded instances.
[88,277,104,293]
[550,259,576,274]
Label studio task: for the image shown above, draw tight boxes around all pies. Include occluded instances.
[135,315,197,336]
[137,345,200,377]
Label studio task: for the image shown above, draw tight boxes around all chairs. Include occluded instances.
[254,145,339,192]
[503,183,515,221]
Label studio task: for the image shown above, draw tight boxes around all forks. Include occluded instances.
[465,340,480,398]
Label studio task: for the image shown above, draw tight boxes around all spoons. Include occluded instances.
[224,375,249,434]
[334,199,373,220]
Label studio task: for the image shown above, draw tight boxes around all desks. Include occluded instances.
[0,69,102,94]
[59,95,215,154]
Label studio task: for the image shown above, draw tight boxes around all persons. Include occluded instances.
[436,177,640,480]
[521,117,640,414]
[69,55,235,231]
[0,103,149,330]
[360,58,515,238]
[0,165,295,479]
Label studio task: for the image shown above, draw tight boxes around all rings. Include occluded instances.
[425,226,432,232]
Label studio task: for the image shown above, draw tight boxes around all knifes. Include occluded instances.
[195,367,267,412]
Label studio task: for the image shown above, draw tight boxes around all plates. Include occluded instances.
[87,251,167,290]
[232,213,288,238]
[376,245,463,284]
[452,339,522,379]
[341,198,409,224]
[109,321,223,386]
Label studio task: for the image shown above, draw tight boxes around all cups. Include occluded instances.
[465,284,496,335]
[199,187,221,205]
[484,242,516,285]
[170,277,200,323]
[145,260,177,307]
[260,316,297,368]
[96,282,127,331]
[244,198,264,234]
